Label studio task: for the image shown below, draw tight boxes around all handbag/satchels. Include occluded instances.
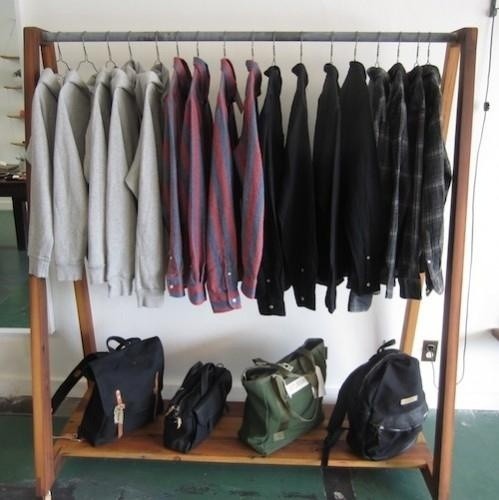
[239,338,327,456]
[165,360,232,454]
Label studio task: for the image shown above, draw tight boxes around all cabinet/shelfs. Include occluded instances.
[18,23,481,500]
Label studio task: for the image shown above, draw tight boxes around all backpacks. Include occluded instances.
[320,339,430,461]
[51,336,164,450]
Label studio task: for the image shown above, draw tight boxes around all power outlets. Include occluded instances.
[421,340,439,361]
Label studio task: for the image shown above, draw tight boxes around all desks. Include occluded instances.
[0,179,29,250]
